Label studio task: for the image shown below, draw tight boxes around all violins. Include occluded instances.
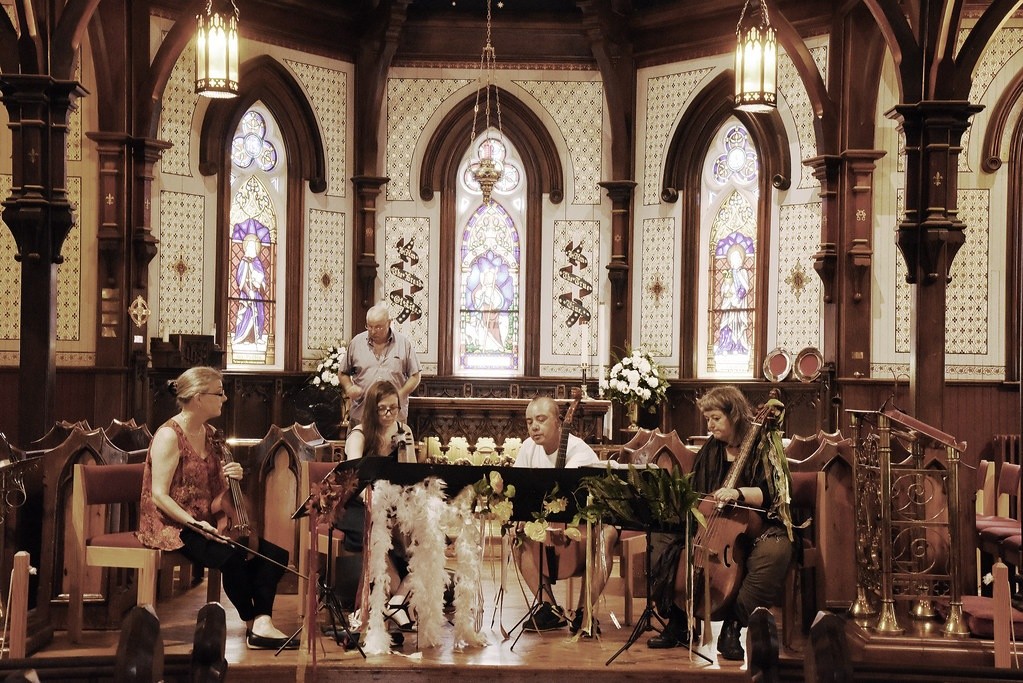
[209,429,259,562]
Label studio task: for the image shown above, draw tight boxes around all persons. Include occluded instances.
[647,385,792,660]
[503,395,620,633]
[343,381,419,633]
[136,367,301,650]
[337,302,422,431]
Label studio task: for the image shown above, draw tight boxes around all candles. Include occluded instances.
[581,320,589,366]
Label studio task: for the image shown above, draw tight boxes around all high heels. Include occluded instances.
[383,605,418,632]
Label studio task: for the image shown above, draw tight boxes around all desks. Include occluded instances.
[409,397,611,445]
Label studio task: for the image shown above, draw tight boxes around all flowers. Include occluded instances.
[598,339,671,415]
[304,338,350,395]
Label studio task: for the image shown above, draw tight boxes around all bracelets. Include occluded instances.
[735,488,745,501]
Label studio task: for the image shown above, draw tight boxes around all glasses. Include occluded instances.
[365,320,388,332]
[376,407,401,417]
[200,390,225,400]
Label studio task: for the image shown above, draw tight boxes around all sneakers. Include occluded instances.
[570,607,601,638]
[522,601,567,632]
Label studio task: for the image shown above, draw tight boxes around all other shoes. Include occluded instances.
[246,628,300,650]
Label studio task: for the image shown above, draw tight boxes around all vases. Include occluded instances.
[627,403,638,430]
[340,391,350,425]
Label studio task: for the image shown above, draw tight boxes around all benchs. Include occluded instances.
[67,464,222,645]
[297,460,345,628]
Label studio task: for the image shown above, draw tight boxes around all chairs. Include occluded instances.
[973,459,1023,568]
[566,530,647,627]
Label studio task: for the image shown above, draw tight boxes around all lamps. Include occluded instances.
[466,0,505,208]
[193,0,242,100]
[732,0,778,113]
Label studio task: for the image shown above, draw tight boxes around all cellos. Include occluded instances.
[671,404,773,622]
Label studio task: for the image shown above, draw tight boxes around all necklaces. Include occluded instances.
[181,414,202,437]
[725,443,740,448]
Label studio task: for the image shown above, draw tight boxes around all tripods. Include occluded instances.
[275,455,370,663]
[484,465,714,665]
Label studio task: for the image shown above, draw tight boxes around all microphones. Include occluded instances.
[878,394,907,417]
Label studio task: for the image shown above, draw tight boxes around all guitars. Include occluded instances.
[531,386,596,580]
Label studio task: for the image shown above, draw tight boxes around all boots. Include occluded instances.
[717,613,744,660]
[647,603,698,648]
